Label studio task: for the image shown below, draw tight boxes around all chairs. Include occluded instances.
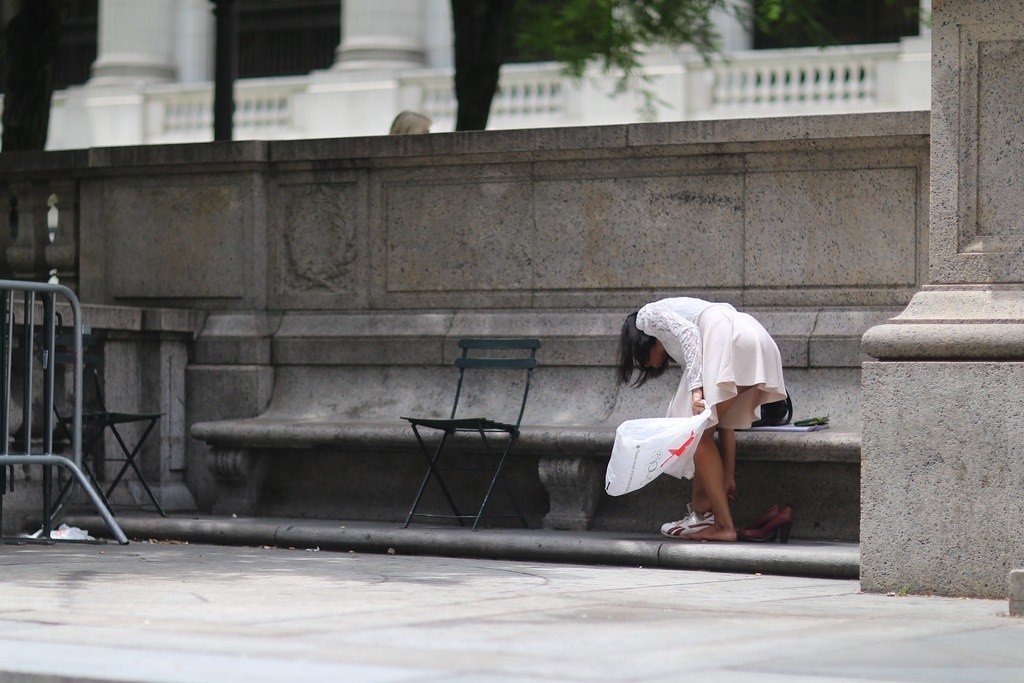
[399,338,540,531]
[37,350,166,517]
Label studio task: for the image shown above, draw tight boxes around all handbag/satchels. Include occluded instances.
[604,399,712,497]
[751,388,793,426]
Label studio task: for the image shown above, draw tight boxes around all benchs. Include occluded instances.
[191,361,861,548]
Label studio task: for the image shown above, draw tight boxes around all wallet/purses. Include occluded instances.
[795,416,828,426]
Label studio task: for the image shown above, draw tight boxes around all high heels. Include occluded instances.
[738,507,780,530]
[740,507,792,543]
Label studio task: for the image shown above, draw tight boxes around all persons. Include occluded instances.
[617,297,787,541]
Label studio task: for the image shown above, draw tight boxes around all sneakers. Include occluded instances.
[660,502,717,537]
